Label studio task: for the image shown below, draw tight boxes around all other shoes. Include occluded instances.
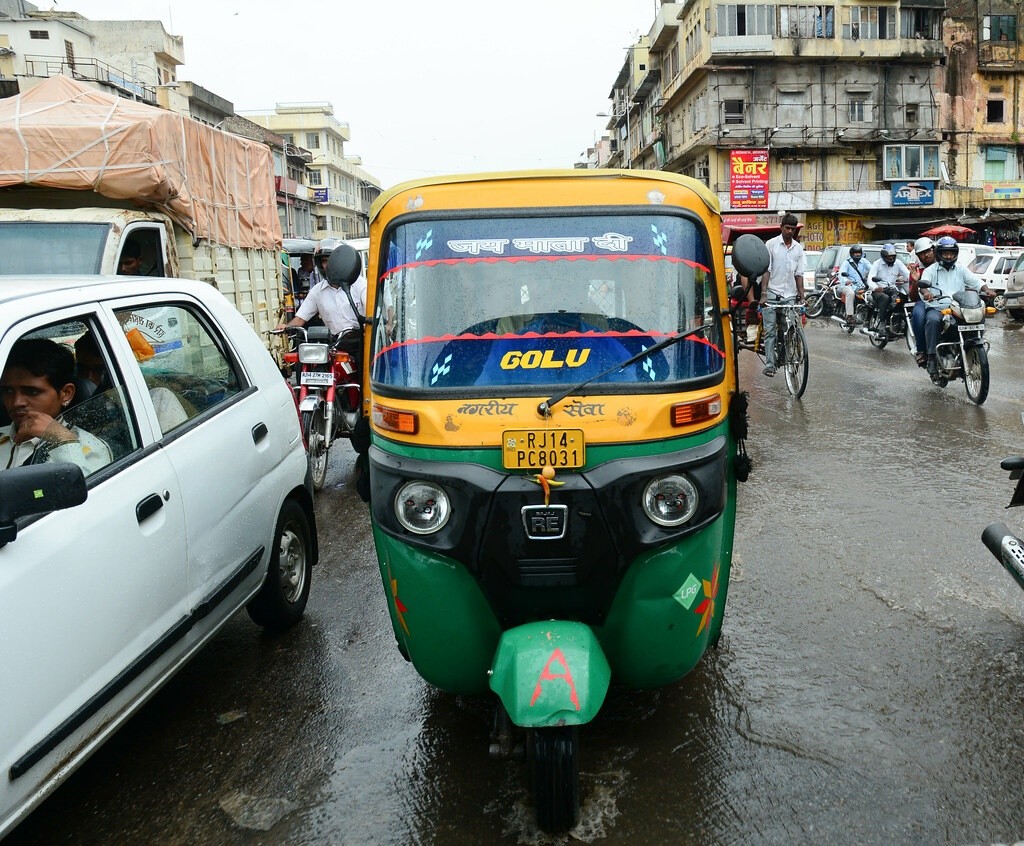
[915,351,925,363]
[847,317,853,323]
[927,362,937,374]
[879,323,885,332]
[764,367,775,377]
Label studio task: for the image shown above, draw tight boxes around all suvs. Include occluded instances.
[814,244,911,291]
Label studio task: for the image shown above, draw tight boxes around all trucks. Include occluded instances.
[0,74,286,380]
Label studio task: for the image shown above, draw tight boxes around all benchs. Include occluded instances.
[731,297,760,307]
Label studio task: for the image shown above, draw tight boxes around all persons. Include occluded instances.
[0,338,113,521]
[273,238,393,374]
[495,263,609,338]
[74,330,188,444]
[733,212,809,375]
[113,237,161,278]
[837,235,997,381]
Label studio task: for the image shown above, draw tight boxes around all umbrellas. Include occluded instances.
[919,224,976,243]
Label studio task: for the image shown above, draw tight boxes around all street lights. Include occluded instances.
[130,57,180,102]
[596,96,631,170]
[282,139,375,238]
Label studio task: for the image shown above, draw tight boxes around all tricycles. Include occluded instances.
[727,285,809,400]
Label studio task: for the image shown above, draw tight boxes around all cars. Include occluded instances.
[0,272,318,846]
[1003,253,1024,322]
[895,242,1024,267]
[342,239,394,280]
[966,253,1021,312]
[803,251,822,297]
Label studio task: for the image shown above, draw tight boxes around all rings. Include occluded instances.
[24,422,27,429]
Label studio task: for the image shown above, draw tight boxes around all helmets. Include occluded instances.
[914,237,934,262]
[849,244,863,260]
[935,236,959,267]
[314,237,346,279]
[880,243,896,264]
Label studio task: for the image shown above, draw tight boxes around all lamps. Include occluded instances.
[876,130,889,138]
[910,129,921,138]
[835,130,844,141]
[768,128,779,141]
[723,129,730,137]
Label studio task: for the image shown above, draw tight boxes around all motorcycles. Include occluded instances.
[280,238,322,373]
[831,272,868,333]
[979,456,1024,591]
[903,280,997,406]
[803,264,840,319]
[859,277,908,349]
[262,324,362,495]
[324,168,772,840]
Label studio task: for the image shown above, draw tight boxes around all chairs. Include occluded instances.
[63,375,225,461]
